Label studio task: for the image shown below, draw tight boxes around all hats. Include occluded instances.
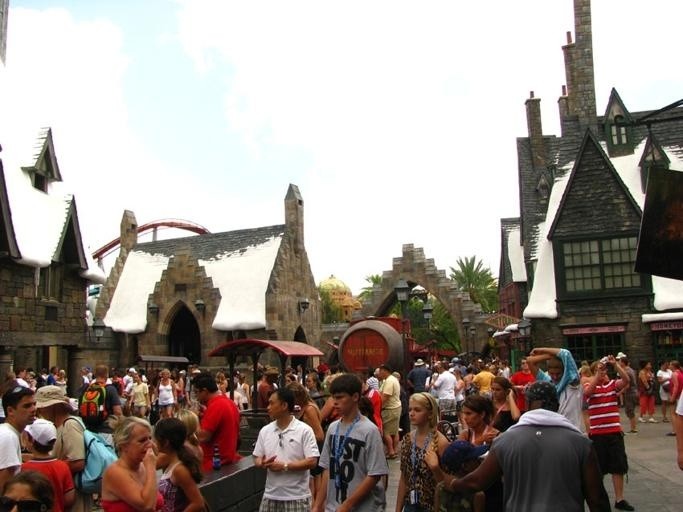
[616,352,627,359]
[414,359,424,366]
[24,419,57,446]
[35,384,69,409]
[442,440,490,465]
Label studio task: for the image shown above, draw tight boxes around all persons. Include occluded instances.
[0,347,683,512]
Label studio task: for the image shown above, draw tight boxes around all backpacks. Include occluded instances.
[73,429,119,494]
[79,383,108,425]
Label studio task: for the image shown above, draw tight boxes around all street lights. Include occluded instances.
[487,327,492,356]
[393,278,410,394]
[461,318,471,360]
[470,325,476,354]
[420,303,433,367]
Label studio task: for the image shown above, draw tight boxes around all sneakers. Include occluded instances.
[625,430,637,435]
[638,417,658,423]
[614,500,633,511]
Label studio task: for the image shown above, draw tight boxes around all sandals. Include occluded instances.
[385,452,398,459]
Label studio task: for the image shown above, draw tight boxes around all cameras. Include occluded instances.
[600,357,610,365]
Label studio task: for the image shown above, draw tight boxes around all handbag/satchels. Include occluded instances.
[663,383,670,392]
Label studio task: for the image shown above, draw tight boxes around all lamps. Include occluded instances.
[195,299,206,318]
[518,321,533,336]
[148,303,159,321]
[298,298,309,313]
